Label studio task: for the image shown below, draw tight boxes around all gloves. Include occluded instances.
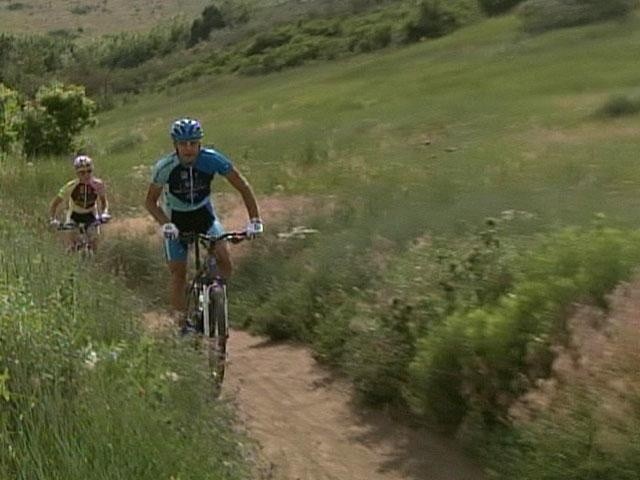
[99,212,113,222]
[244,215,264,241]
[50,218,61,227]
[160,222,182,241]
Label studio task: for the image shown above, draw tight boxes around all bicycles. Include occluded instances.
[53,218,110,258]
[178,228,260,398]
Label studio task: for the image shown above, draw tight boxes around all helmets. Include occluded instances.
[169,116,206,141]
[72,154,95,175]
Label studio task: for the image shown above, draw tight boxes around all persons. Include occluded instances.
[47,155,112,253]
[143,118,264,337]
